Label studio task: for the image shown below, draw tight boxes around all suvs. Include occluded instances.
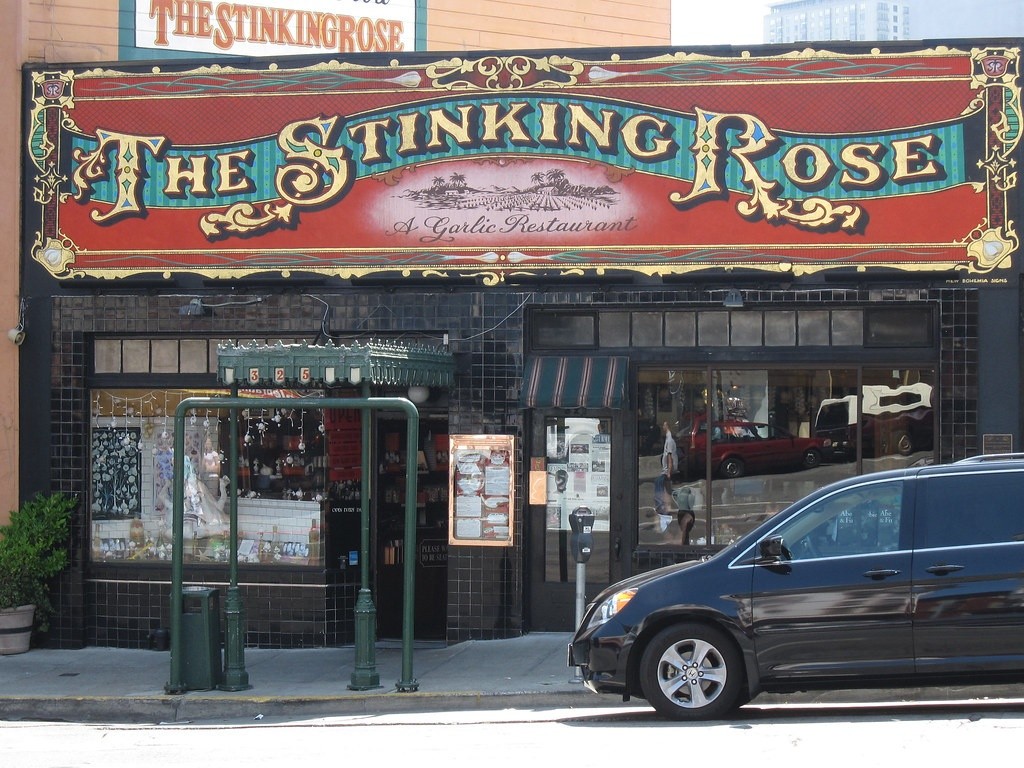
[814,381,935,457]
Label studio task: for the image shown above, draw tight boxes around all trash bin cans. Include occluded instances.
[169,585,222,693]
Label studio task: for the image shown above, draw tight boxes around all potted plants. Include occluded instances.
[0,489,80,654]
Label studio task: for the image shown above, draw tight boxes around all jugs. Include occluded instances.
[688,487,705,510]
[672,486,688,510]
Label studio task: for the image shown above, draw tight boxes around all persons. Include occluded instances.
[663,421,678,495]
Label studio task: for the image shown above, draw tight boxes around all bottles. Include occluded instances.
[155,527,168,560]
[722,487,730,504]
[145,531,155,559]
[308,519,320,565]
[192,530,201,562]
[130,512,146,557]
[93,524,102,562]
[270,526,283,560]
[257,532,266,563]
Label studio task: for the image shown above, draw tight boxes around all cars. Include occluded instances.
[679,412,826,480]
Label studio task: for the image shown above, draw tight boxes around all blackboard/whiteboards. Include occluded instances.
[92,426,142,521]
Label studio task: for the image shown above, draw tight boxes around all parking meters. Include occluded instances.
[567,506,596,683]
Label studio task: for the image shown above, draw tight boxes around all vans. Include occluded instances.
[564,452,1024,723]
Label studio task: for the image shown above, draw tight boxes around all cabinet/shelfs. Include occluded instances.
[221,408,449,639]
